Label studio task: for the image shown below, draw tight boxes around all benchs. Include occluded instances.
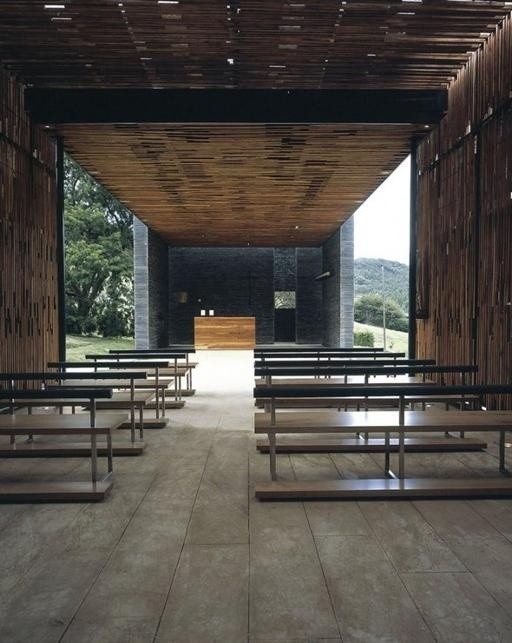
[0,348,199,502]
[253,345,512,502]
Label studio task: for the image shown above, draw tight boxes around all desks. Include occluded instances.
[193,316,256,351]
